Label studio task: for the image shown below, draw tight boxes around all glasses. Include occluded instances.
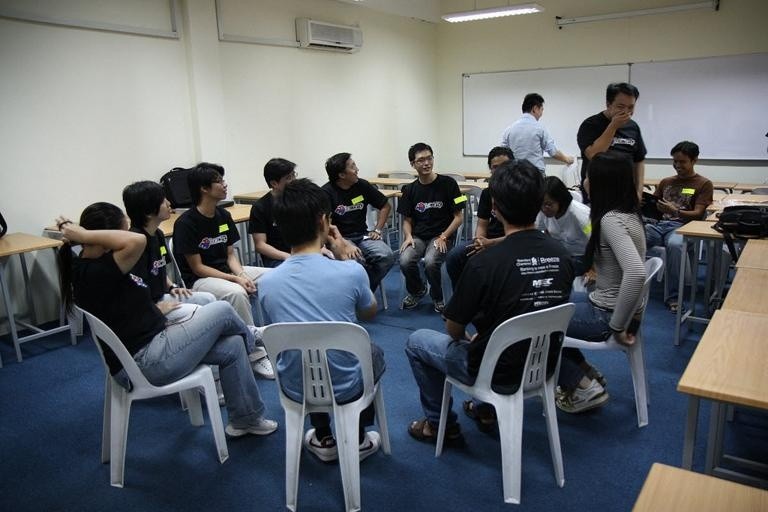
[286,171,298,180]
[413,155,434,165]
[211,179,225,184]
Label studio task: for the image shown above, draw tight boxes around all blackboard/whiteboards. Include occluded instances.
[462,51,768,160]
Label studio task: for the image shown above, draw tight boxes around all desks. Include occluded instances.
[630,183,766,511]
[1,233,78,361]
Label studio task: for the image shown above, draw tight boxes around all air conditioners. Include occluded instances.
[293,16,363,55]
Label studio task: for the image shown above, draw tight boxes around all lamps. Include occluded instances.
[438,1,546,24]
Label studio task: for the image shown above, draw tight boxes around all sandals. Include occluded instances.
[668,298,687,314]
[407,415,461,443]
[462,397,500,434]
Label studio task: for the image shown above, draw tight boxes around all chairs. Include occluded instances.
[262,321,390,511]
[554,257,664,429]
[46,164,707,410]
[73,303,229,488]
[435,303,576,504]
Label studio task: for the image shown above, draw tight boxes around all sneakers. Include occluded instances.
[401,283,446,313]
[244,324,276,380]
[224,417,279,437]
[555,365,611,414]
[304,426,383,463]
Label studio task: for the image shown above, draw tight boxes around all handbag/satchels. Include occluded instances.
[711,204,768,263]
[160,161,225,209]
